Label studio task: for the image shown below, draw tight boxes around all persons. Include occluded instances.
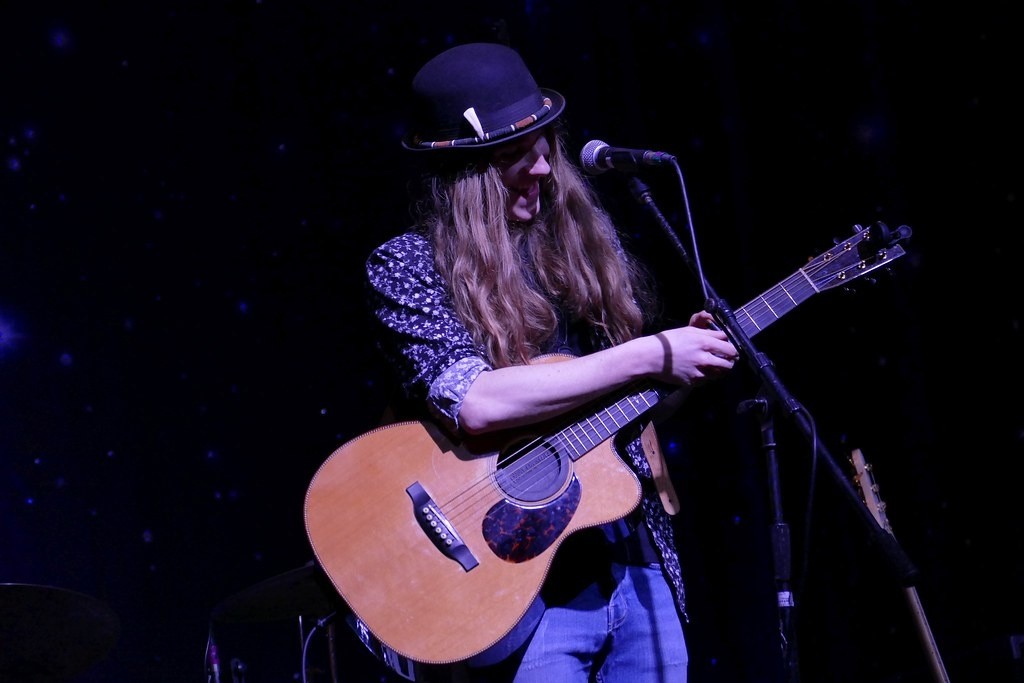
[363,42,738,683]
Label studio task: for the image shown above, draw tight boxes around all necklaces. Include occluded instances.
[547,313,576,355]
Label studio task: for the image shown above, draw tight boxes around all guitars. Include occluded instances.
[302,217,916,683]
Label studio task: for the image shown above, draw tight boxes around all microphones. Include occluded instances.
[581,140,677,175]
[209,636,220,683]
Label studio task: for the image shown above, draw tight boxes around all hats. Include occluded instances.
[397,42,568,154]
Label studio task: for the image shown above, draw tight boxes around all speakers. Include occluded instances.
[202,563,415,683]
